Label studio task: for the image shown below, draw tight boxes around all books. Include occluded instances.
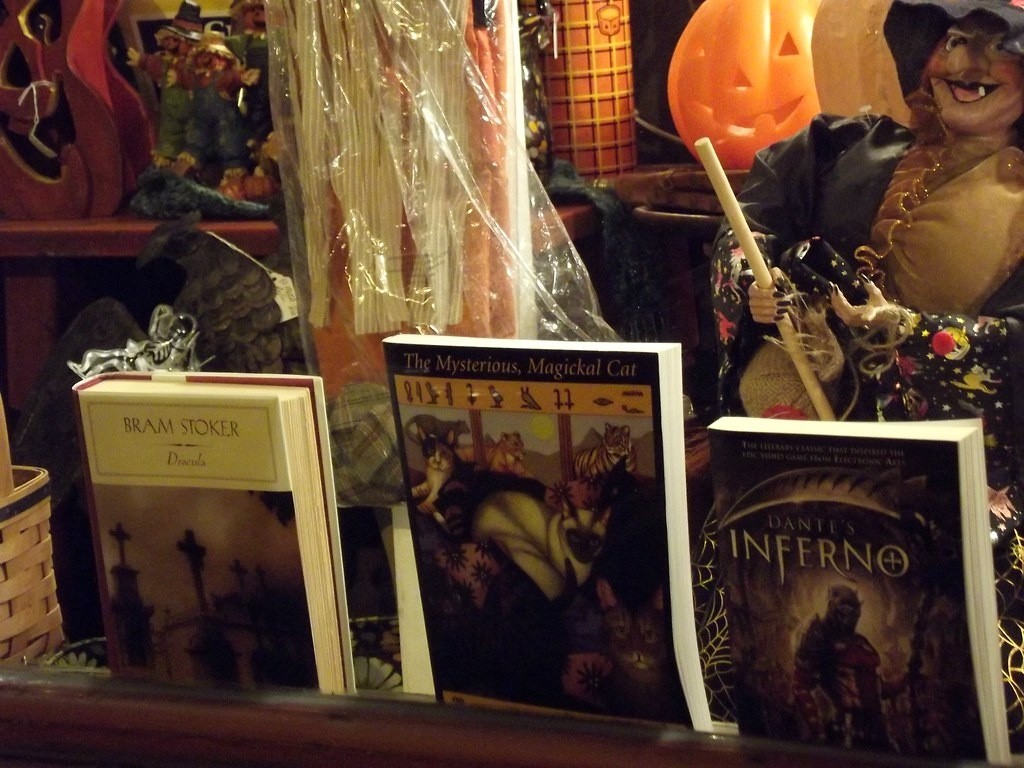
[68,370,374,695]
[378,331,714,738]
[708,412,1014,765]
